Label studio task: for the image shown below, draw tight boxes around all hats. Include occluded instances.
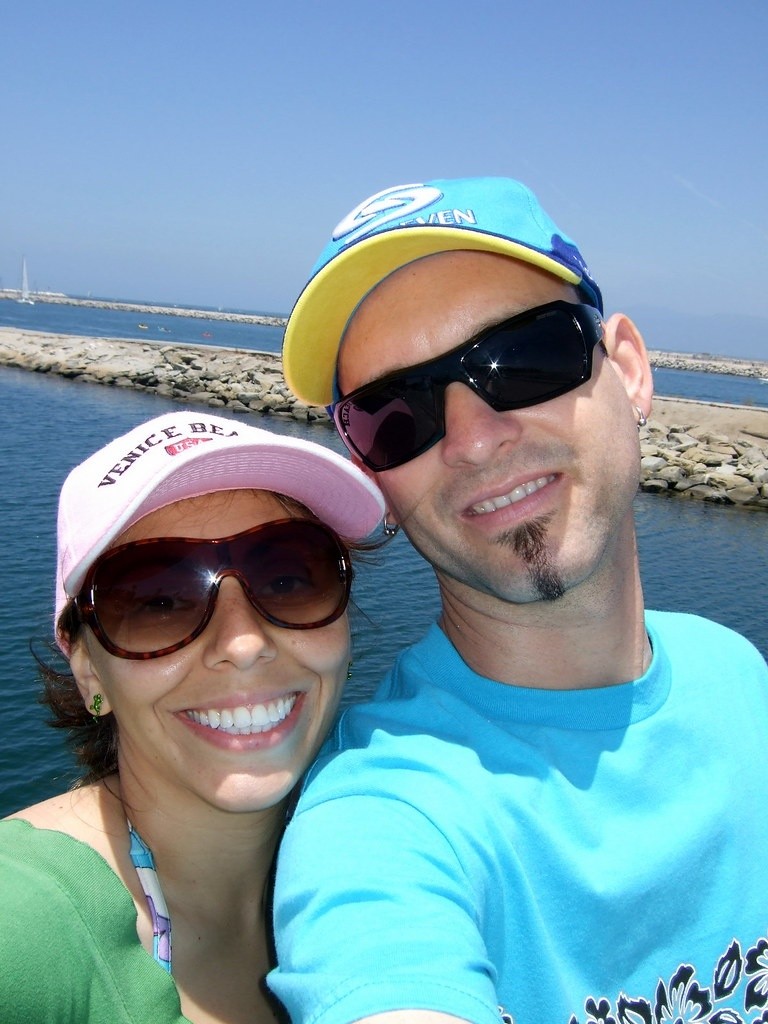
[282,177,604,424]
[54,410,385,662]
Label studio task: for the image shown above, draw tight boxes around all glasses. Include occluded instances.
[67,515,352,661]
[333,299,604,472]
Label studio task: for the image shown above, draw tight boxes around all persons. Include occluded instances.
[266,177,768,1024]
[0,411,386,1024]
